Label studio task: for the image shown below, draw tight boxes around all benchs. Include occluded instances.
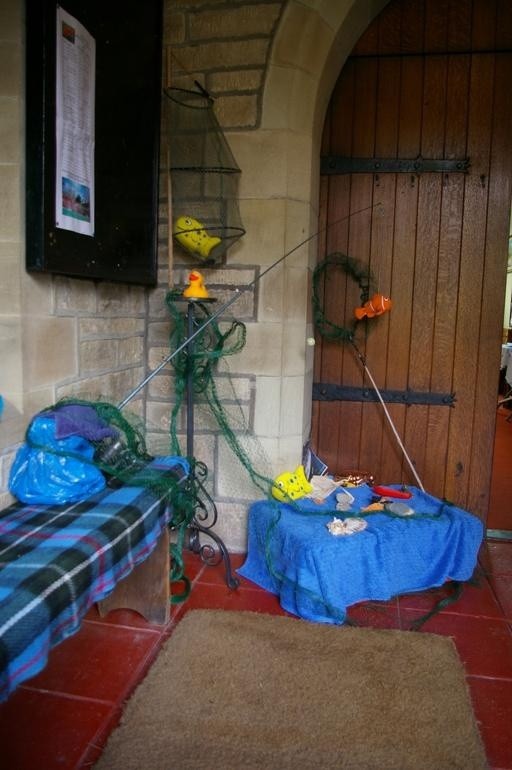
[0,455,192,713]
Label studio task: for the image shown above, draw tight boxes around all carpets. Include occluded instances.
[89,608,487,770]
